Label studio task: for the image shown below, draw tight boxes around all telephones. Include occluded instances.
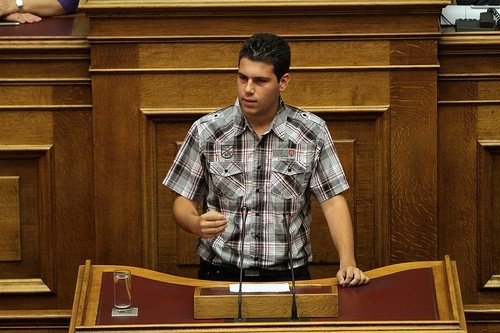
[455,7,500,31]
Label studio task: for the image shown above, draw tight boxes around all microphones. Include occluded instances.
[237,206,248,322]
[284,211,298,320]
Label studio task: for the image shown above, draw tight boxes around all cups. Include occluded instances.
[113,269,132,309]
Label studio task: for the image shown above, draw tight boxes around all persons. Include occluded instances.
[161,33,369,290]
[0,0,80,23]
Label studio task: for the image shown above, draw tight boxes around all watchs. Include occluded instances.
[16,0,24,14]
